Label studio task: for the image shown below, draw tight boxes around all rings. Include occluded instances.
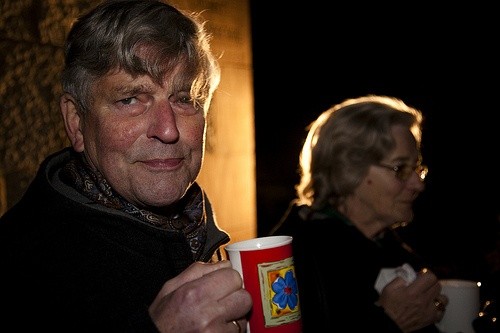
[433,298,441,310]
[232,320,241,333]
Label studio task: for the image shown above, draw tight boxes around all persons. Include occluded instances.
[265,95,450,333]
[0,0,253,333]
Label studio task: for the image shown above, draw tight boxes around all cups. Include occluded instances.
[224,236,303,333]
[433,279,481,332]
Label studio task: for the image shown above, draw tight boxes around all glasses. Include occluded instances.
[370,161,429,181]
